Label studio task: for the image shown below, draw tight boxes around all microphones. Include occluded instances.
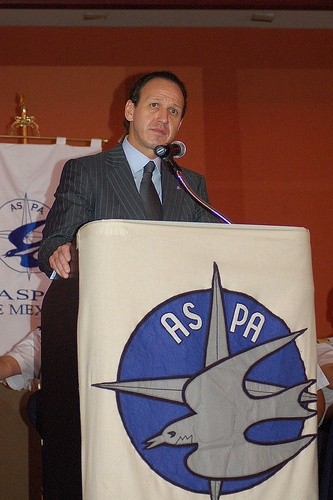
[154,141,186,158]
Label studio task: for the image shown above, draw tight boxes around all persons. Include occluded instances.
[1,324,41,391]
[315,363,330,429]
[38,71,220,478]
[315,335,333,430]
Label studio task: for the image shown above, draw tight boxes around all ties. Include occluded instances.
[140,161,163,221]
